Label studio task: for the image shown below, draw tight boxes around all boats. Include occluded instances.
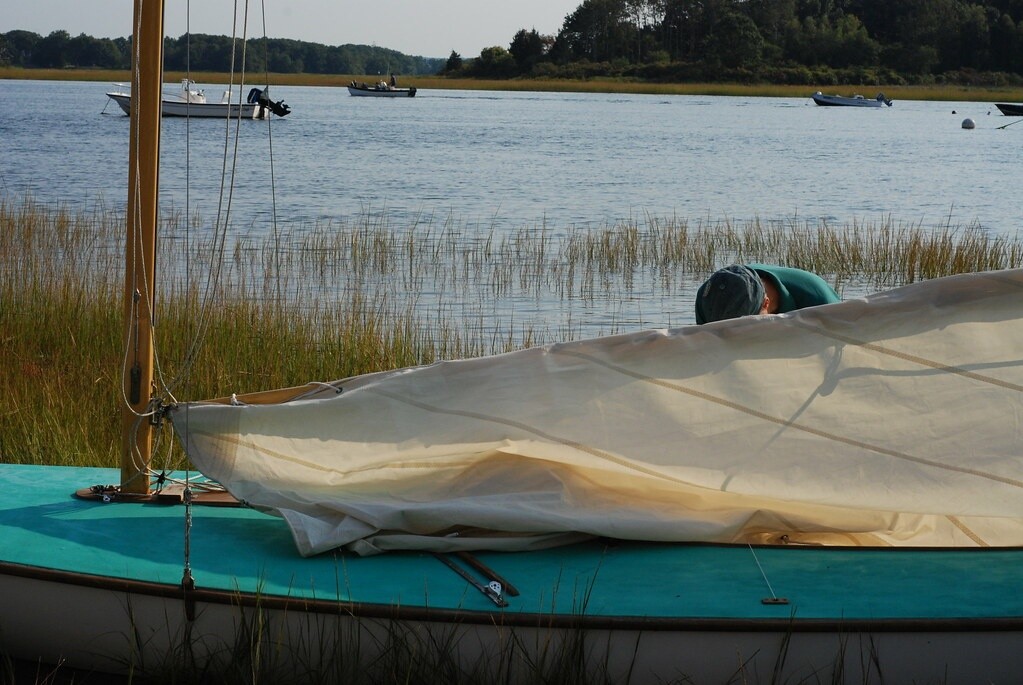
[995,104,1023,116]
[105,82,291,119]
[812,91,893,107]
[347,85,416,97]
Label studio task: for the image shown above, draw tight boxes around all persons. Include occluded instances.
[694,265,842,326]
[350,73,398,91]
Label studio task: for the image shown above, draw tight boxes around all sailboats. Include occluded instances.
[0,0,1022,685]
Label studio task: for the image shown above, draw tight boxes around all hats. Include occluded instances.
[702,264,765,322]
